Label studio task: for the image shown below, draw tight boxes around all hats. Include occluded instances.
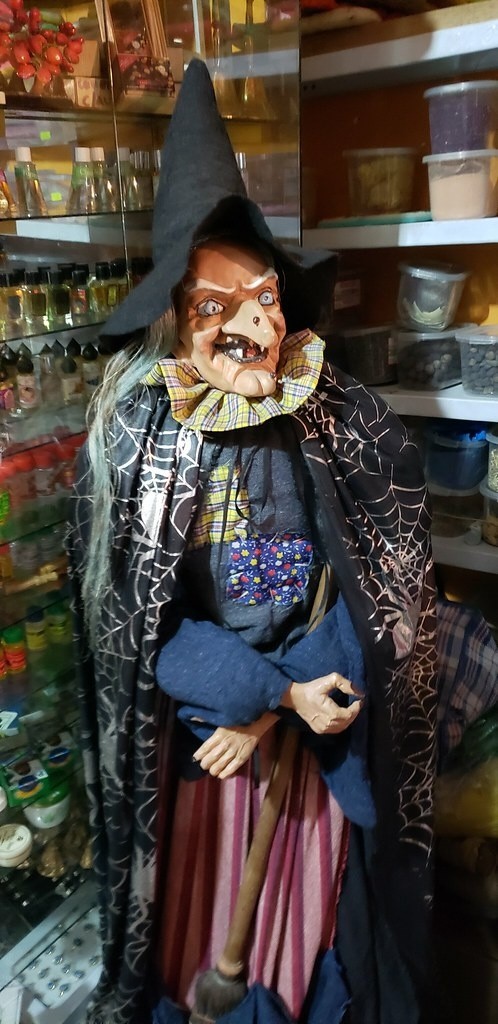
[98,58,340,353]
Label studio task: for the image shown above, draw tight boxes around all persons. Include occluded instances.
[61,57,441,1024]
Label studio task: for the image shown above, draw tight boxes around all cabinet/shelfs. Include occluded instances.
[0,0,498,1024]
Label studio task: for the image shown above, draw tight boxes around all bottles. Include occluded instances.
[0,432,93,538]
[211,20,281,121]
[0,590,76,682]
[0,256,157,325]
[0,337,125,450]
[0,142,298,218]
[0,519,68,585]
